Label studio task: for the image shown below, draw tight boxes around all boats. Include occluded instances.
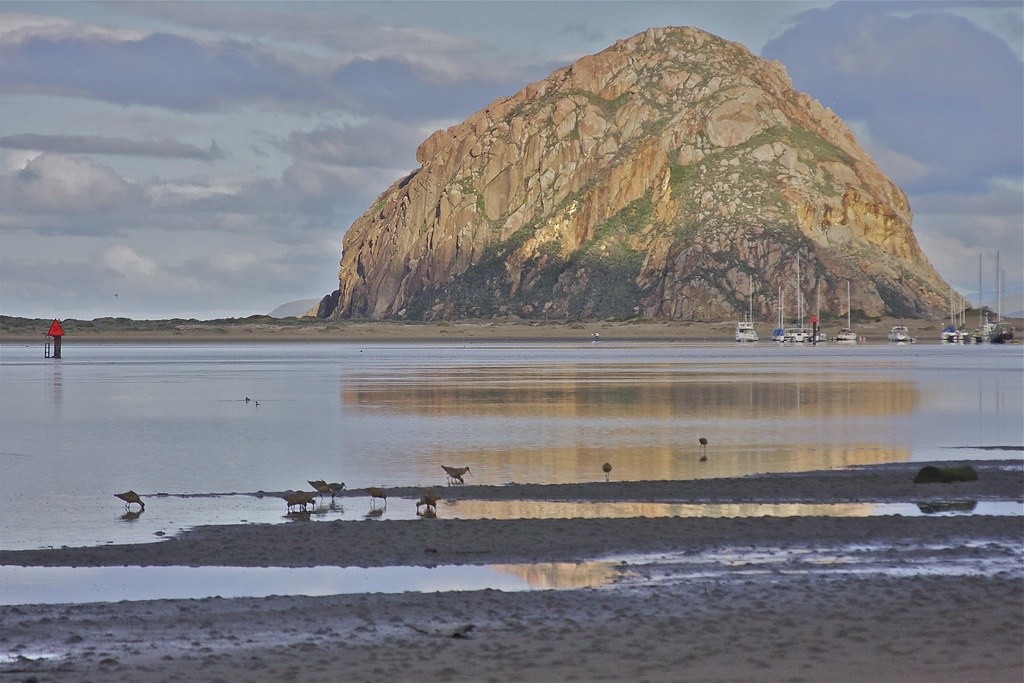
[887,326,912,341]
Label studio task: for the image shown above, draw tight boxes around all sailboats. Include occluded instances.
[773,250,827,343]
[735,275,759,341]
[832,281,858,341]
[941,250,1012,342]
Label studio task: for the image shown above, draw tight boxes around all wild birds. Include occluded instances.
[307,479,346,500]
[361,487,387,503]
[416,495,441,510]
[603,463,612,475]
[281,493,317,509]
[114,491,145,509]
[699,438,708,447]
[441,465,470,484]
